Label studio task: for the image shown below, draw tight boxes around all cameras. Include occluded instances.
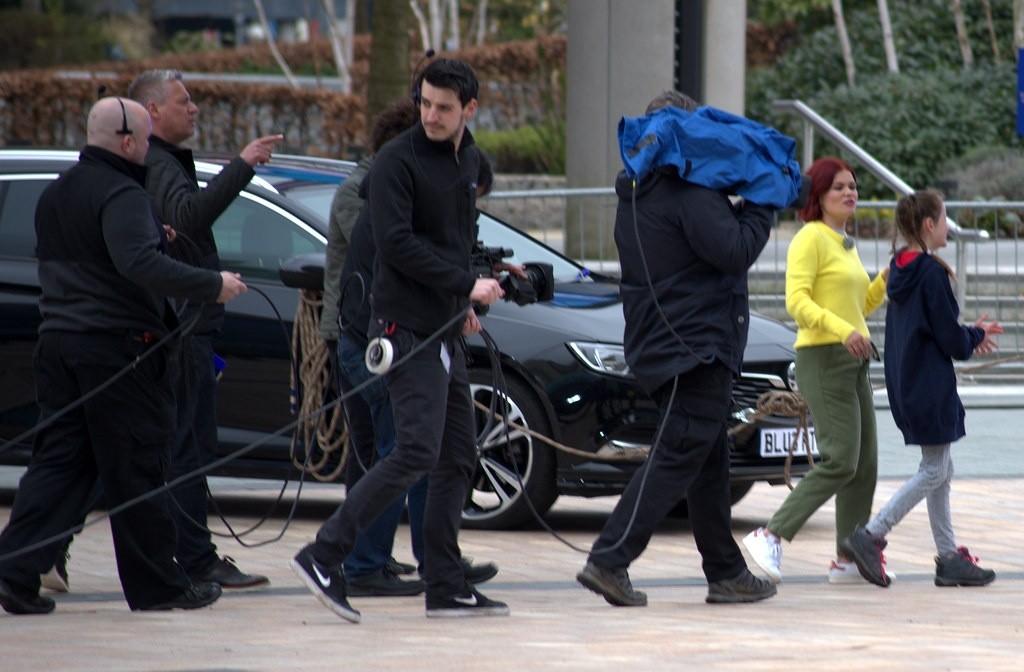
[498,260,555,306]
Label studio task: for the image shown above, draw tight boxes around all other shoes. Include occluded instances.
[389,558,419,574]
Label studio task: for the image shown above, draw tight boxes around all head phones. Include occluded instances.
[412,57,479,106]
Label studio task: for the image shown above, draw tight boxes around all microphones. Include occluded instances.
[97,84,106,100]
[413,47,435,81]
[842,237,854,250]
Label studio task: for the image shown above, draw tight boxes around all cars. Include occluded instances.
[0,146,821,530]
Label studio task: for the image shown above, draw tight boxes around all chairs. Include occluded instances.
[242,192,293,271]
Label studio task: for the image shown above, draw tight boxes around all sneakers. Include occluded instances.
[742,527,783,580]
[577,559,648,606]
[933,546,995,586]
[706,567,778,604]
[0,574,55,612]
[829,560,896,584]
[845,523,892,587]
[427,580,509,619]
[290,546,362,624]
[142,580,222,610]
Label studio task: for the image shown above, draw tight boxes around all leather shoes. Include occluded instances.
[195,556,267,588]
[345,564,424,596]
[40,555,70,591]
[455,555,499,584]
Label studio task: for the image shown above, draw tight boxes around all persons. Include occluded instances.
[0,71,283,616]
[575,90,776,607]
[740,158,896,585]
[839,191,1004,587]
[290,56,529,626]
